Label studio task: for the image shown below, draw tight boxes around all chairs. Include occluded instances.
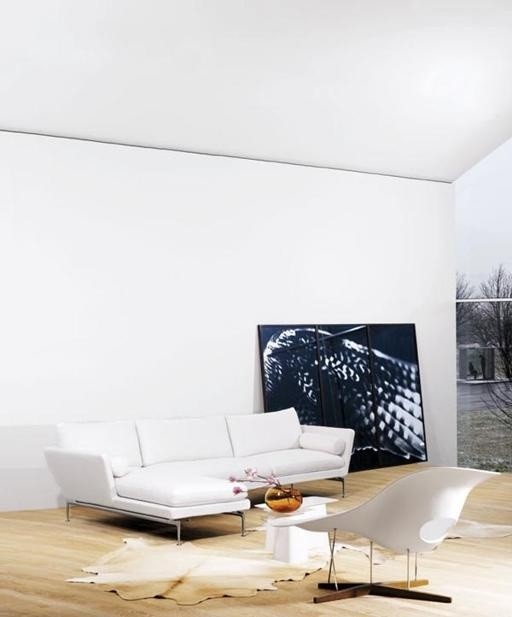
[266,466,503,604]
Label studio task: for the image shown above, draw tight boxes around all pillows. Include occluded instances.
[135,415,234,467]
[59,420,143,469]
[298,432,346,455]
[224,407,302,457]
[109,457,131,477]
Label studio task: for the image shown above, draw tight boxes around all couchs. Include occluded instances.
[43,407,356,545]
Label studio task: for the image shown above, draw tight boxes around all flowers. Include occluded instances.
[228,466,302,504]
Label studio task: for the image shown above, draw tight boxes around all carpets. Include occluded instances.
[64,524,389,606]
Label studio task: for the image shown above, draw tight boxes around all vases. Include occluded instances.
[265,486,303,513]
